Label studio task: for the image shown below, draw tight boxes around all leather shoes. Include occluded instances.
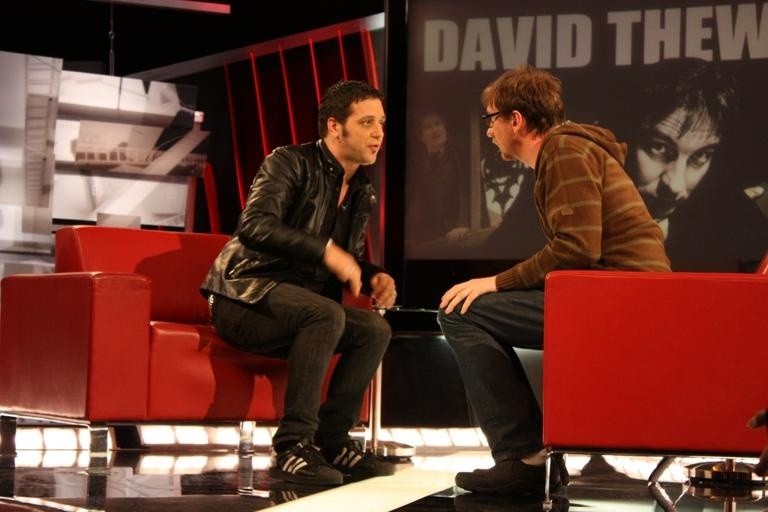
[473,459,569,486]
[454,461,562,495]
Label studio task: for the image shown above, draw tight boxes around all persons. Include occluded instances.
[614,54,767,275]
[401,107,470,260]
[199,80,397,486]
[436,64,672,499]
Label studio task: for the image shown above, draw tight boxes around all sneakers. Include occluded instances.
[315,437,394,477]
[267,438,344,486]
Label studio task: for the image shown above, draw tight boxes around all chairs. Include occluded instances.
[542,254,768,512]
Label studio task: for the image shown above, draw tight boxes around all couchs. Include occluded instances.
[0,225,372,451]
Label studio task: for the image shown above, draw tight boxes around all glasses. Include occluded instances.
[479,110,502,128]
